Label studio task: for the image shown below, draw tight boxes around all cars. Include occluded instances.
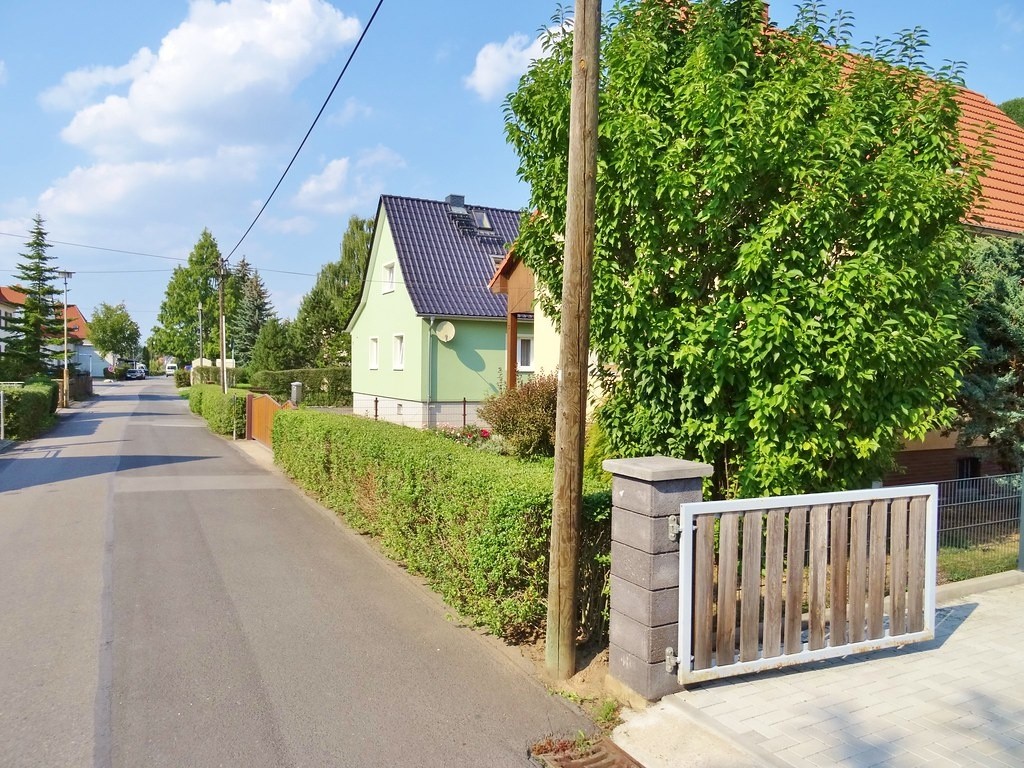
[126,363,148,380]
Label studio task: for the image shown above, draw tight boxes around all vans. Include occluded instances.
[165,364,178,377]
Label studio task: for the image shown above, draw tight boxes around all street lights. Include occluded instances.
[56,266,76,409]
